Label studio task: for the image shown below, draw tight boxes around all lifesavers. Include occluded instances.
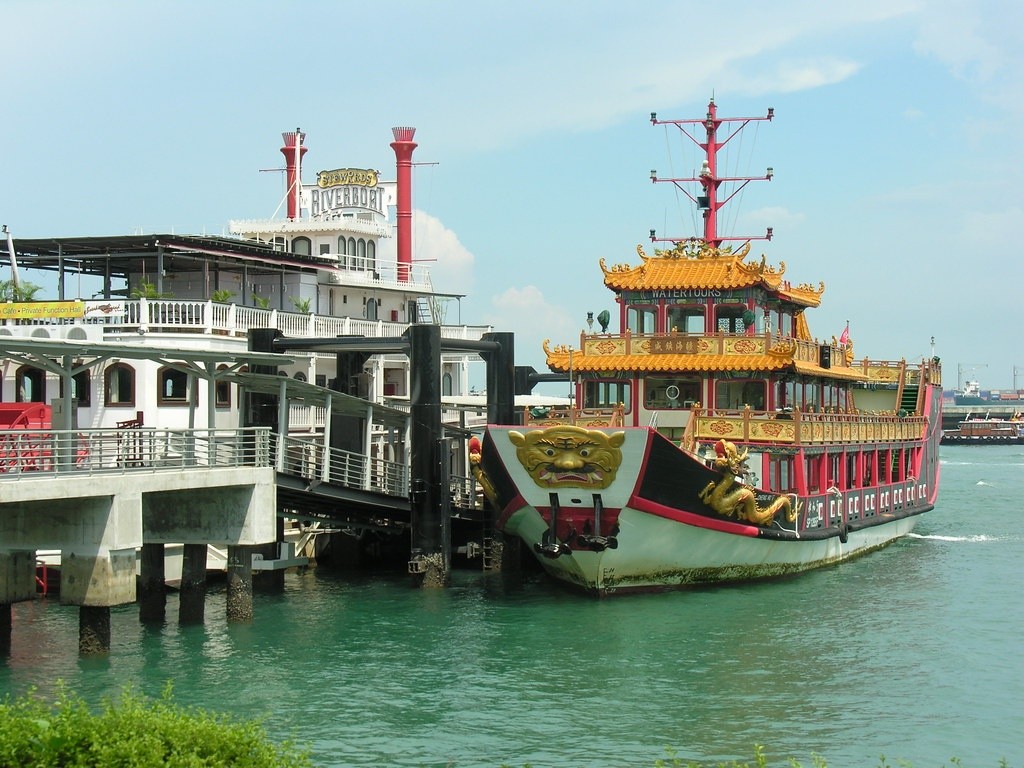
[999,436,1004,441]
[839,523,848,543]
[978,435,984,441]
[986,436,992,442]
[966,435,971,442]
[949,435,954,441]
[956,436,962,442]
[993,435,997,442]
[665,385,680,400]
[942,436,946,441]
[1005,435,1011,443]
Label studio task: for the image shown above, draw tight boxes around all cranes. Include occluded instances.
[956,362,988,390]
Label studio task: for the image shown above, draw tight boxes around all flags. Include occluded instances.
[840,326,848,343]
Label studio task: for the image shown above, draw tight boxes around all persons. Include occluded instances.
[862,480,871,486]
[852,480,856,488]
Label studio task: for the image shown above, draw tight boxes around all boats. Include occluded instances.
[939,406,1024,446]
[466,86,946,603]
[0,123,576,596]
